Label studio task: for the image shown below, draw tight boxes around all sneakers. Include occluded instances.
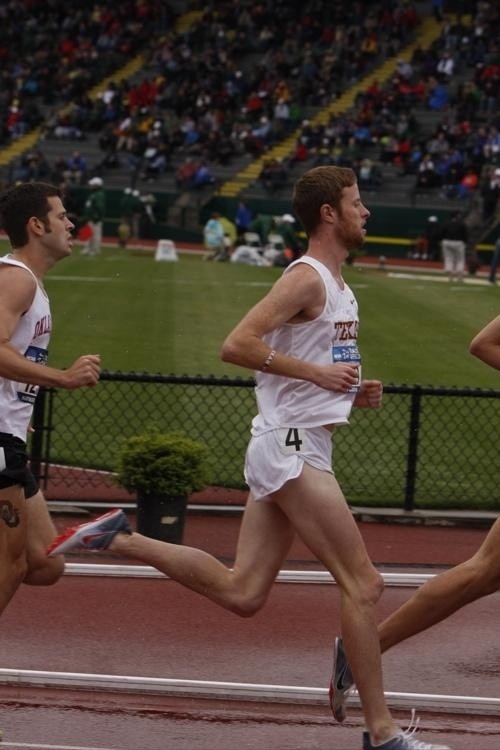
[328,636,357,723]
[43,508,132,560]
[362,728,454,750]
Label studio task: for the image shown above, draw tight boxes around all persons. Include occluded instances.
[328,313,499,722]
[48,167,454,750]
[1,182,102,619]
[2,1,500,283]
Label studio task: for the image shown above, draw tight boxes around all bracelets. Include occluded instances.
[262,349,277,370]
